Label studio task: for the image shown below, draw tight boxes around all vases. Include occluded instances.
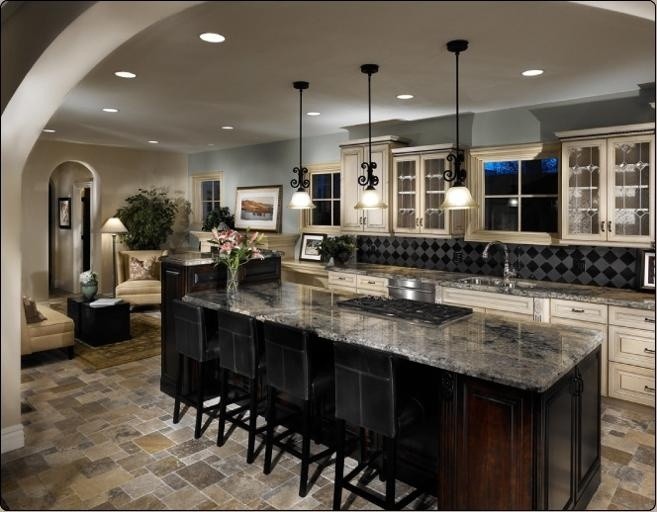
[79,280,98,301]
[225,266,241,301]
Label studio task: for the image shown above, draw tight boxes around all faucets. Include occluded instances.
[481,240,517,293]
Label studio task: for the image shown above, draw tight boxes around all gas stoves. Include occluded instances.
[335,296,474,327]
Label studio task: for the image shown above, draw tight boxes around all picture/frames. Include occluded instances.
[57,196,71,230]
[233,184,283,234]
[635,248,656,293]
[298,232,328,263]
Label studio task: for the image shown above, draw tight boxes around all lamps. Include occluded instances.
[98,217,130,298]
[438,38,480,211]
[286,80,318,211]
[353,62,390,211]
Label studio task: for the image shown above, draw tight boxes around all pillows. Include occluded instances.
[22,296,48,324]
[128,253,156,280]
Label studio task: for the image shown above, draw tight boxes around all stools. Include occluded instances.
[66,293,131,347]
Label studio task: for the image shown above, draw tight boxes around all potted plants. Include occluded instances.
[112,186,179,279]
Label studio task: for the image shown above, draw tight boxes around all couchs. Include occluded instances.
[115,249,169,305]
[21,293,76,360]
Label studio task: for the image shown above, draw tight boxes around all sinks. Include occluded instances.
[458,276,535,292]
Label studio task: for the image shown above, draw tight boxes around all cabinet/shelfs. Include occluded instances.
[555,122,657,249]
[549,299,655,408]
[327,271,390,298]
[434,285,533,320]
[340,134,410,237]
[160,257,281,403]
[390,142,466,239]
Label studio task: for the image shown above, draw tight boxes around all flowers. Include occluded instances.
[78,270,97,284]
[206,221,266,295]
